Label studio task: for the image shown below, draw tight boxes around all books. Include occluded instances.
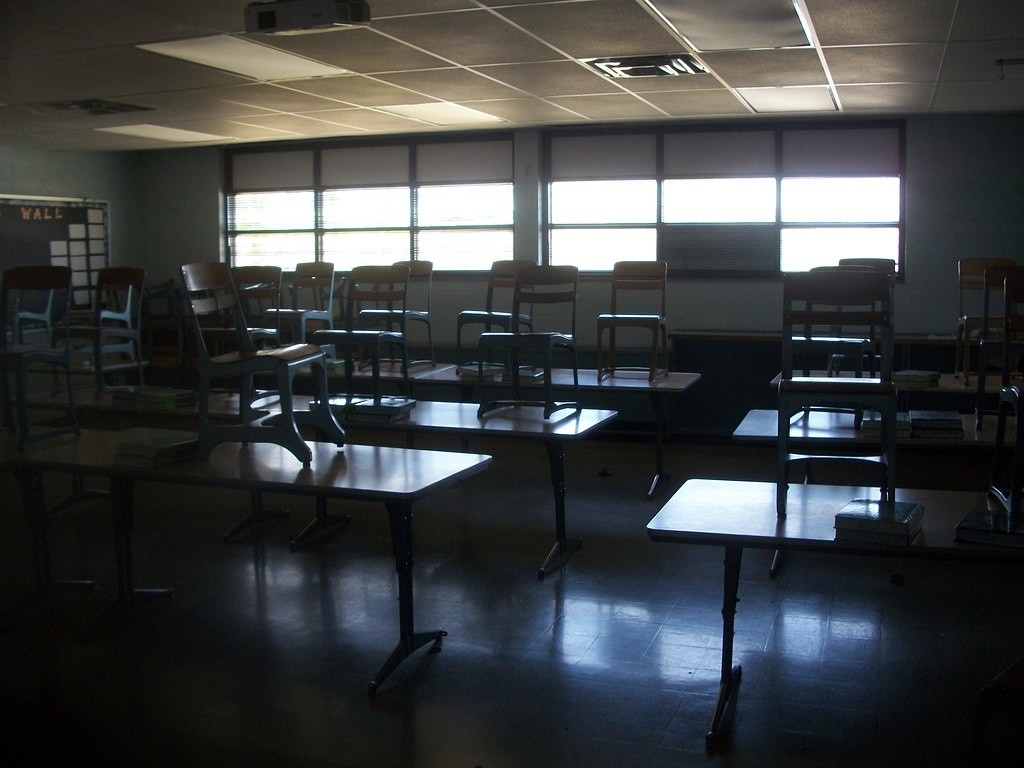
[890,369,940,388]
[954,508,1024,549]
[109,385,197,415]
[861,410,963,440]
[323,357,346,371]
[111,434,199,467]
[832,500,924,548]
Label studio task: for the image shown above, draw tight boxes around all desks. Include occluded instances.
[0,350,1024,749]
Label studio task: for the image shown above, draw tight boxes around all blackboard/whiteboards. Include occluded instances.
[0,193,113,332]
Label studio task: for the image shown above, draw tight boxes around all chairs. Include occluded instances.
[0,257,1024,519]
